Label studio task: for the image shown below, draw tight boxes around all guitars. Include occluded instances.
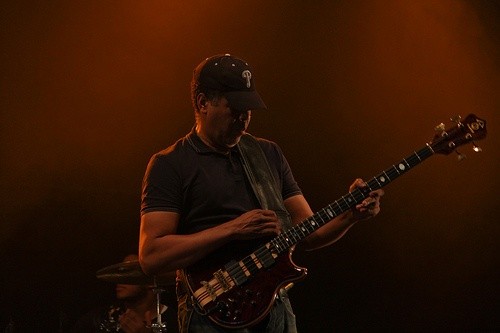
[183,113,488,329]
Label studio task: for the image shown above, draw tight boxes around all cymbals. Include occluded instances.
[95,260,175,284]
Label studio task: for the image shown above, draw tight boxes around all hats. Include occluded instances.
[193,52,267,110]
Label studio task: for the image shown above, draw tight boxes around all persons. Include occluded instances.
[136,52,386,333]
[95,242,167,333]
